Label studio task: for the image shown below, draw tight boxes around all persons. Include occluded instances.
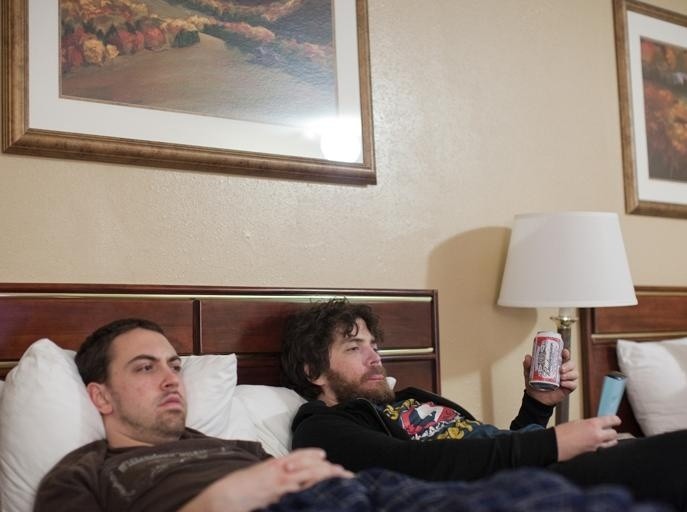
[280,295,687,511]
[35,317,675,511]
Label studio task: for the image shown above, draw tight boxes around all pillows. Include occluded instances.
[234,374,396,455]
[614,339,685,435]
[3,338,237,509]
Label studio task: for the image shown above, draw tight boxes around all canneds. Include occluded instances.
[528,330,564,391]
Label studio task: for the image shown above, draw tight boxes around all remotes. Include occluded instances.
[598,371,628,416]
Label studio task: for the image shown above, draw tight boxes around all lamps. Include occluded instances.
[496,211,638,426]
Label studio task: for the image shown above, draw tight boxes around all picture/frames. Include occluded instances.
[2,0,377,189]
[613,1,687,218]
